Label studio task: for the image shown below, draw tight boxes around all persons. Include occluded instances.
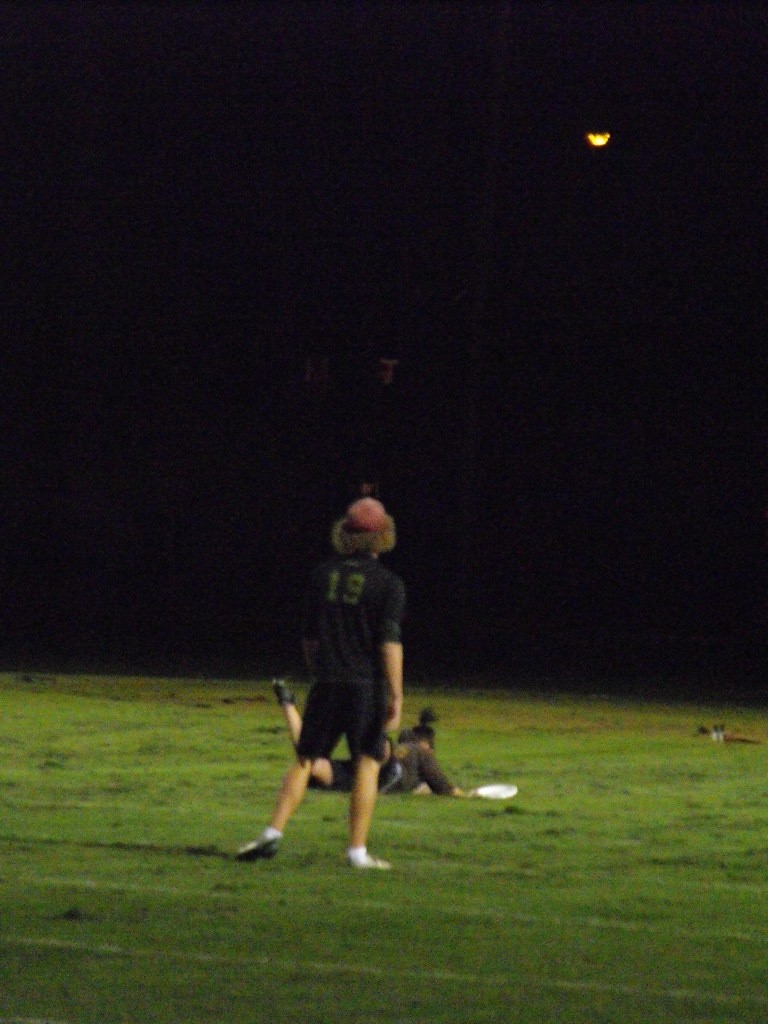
[273,679,475,798]
[236,497,403,867]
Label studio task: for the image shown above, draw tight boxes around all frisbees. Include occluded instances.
[477,784,517,799]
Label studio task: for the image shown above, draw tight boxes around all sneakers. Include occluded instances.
[233,836,279,861]
[346,853,394,872]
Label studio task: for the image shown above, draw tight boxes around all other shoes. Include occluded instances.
[272,679,295,704]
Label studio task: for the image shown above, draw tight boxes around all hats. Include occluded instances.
[349,498,384,532]
